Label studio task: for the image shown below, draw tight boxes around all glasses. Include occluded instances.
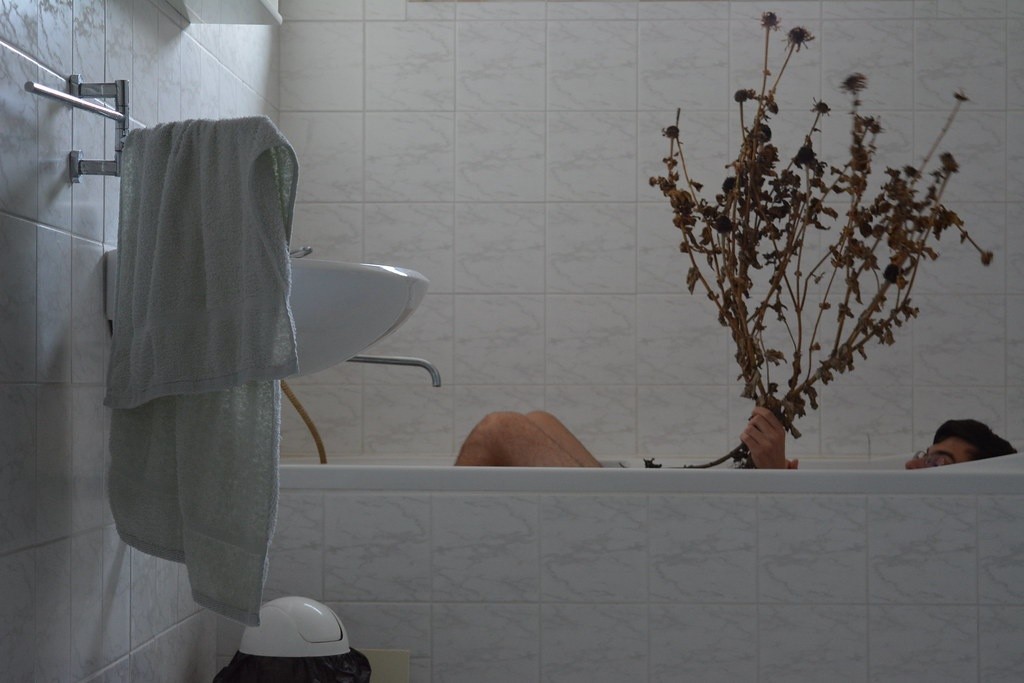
[913,451,954,467]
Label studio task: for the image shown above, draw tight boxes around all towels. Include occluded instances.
[100,116,307,631]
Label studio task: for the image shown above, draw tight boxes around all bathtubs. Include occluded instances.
[217,441,1024,683]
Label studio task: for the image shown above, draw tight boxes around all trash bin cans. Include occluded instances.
[212,595,373,683]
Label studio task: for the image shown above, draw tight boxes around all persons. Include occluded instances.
[449,410,1018,469]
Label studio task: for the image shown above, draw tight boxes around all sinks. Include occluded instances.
[105,244,431,385]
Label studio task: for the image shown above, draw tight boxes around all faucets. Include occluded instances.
[343,353,443,390]
